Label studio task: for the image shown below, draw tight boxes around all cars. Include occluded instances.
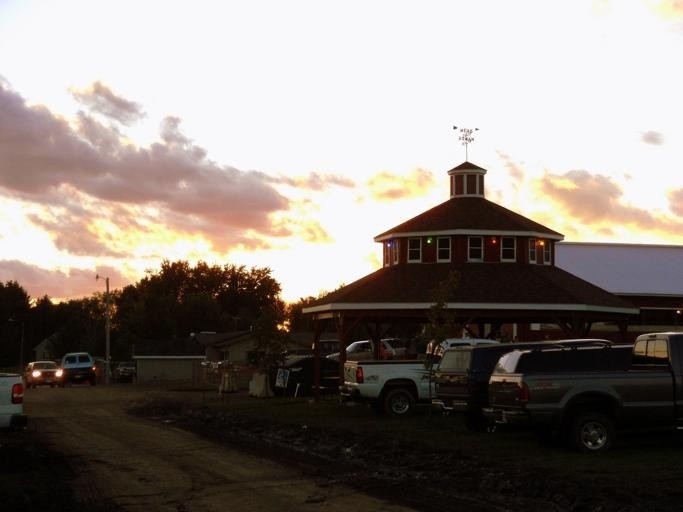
[24,352,136,389]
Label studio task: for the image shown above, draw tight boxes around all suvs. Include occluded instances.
[432,338,615,411]
[325,339,409,363]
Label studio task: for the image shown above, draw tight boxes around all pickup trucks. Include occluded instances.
[342,338,501,420]
[0,373,28,433]
[488,332,683,457]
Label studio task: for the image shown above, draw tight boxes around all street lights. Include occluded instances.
[95,274,113,378]
